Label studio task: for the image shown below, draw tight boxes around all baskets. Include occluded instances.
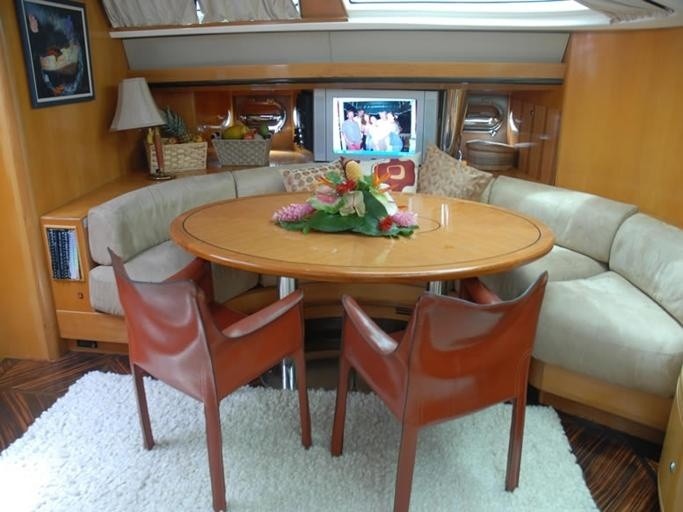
[146,141,207,175]
[212,139,270,166]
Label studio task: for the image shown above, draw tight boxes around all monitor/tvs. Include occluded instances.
[311,87,440,165]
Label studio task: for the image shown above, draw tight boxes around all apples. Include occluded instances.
[165,137,177,144]
[192,136,202,143]
[241,132,253,139]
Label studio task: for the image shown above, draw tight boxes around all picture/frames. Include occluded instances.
[13,0,95,108]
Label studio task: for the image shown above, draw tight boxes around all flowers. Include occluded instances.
[268,159,419,240]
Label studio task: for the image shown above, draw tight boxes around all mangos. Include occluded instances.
[222,125,249,139]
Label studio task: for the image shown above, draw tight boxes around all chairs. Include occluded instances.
[107,248,312,512]
[331,271,550,512]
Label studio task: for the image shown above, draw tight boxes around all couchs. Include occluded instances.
[87,166,682,439]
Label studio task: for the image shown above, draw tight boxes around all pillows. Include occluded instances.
[279,158,348,192]
[342,153,420,194]
[418,137,492,200]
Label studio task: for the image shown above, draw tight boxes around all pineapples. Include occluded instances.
[157,102,193,144]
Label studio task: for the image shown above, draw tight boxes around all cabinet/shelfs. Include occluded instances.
[656,369,683,512]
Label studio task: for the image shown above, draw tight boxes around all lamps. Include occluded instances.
[108,77,176,180]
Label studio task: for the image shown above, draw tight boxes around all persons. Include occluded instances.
[342,108,403,151]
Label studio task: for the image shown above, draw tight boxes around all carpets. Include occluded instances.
[0,369,600,512]
